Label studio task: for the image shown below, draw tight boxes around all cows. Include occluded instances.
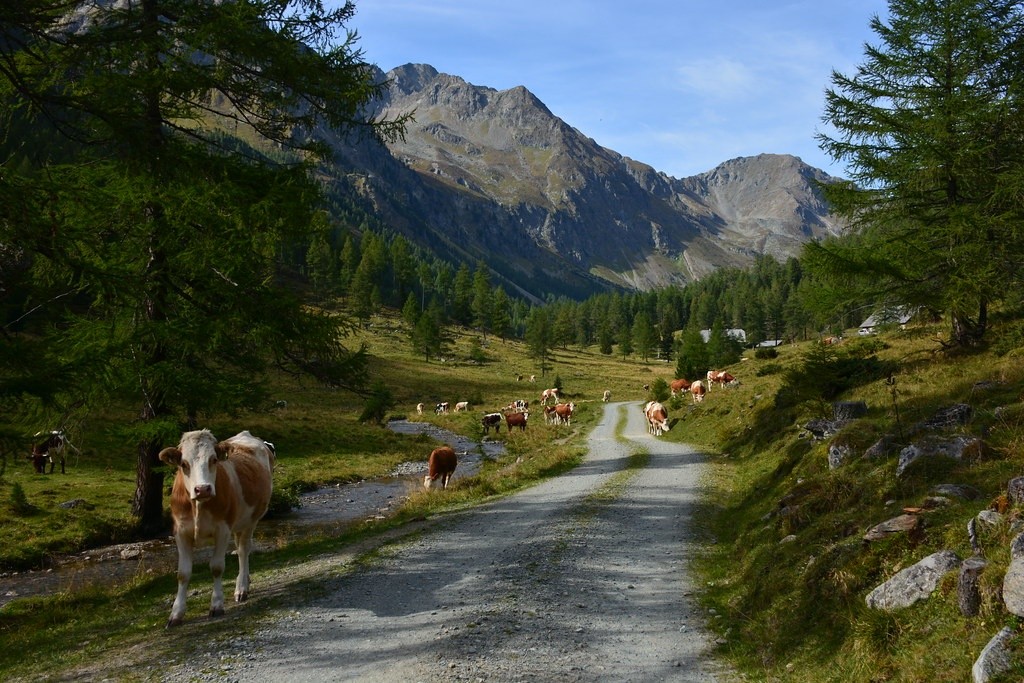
[516,374,524,383]
[671,370,737,405]
[602,390,611,402]
[454,401,468,414]
[434,401,451,415]
[643,384,650,393]
[417,402,424,416]
[643,400,670,437]
[421,446,458,493]
[538,386,577,428]
[481,398,531,438]
[530,375,537,385]
[26,429,83,477]
[158,427,277,629]
